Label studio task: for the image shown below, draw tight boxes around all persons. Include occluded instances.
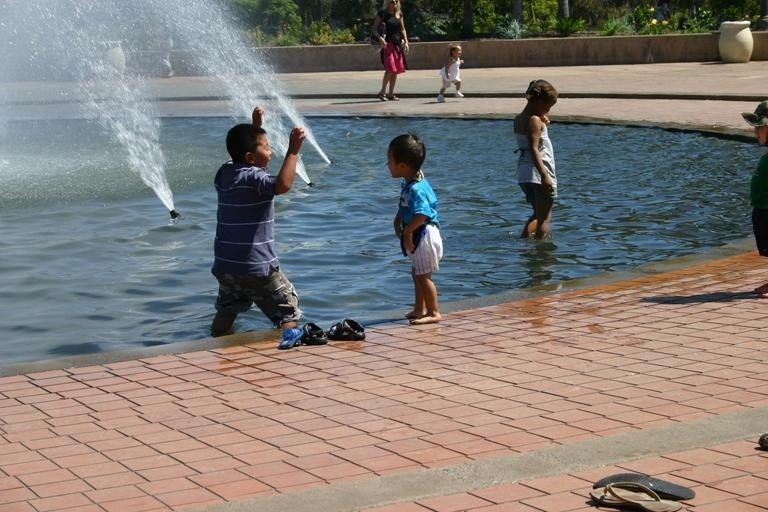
[437,44,464,102]
[740,99,768,296]
[210,107,307,336]
[145,14,174,77]
[512,79,558,239]
[385,131,443,325]
[372,0,409,101]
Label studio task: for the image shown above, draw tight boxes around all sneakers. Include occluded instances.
[437,96,446,103]
[455,92,465,99]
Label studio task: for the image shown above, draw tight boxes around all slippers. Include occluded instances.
[278,327,304,349]
[593,472,695,500]
[589,480,683,512]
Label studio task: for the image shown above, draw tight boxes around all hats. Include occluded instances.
[740,100,768,128]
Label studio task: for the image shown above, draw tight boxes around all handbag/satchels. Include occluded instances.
[369,11,387,48]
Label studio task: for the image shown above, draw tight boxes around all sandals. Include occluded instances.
[301,322,327,345]
[378,93,389,102]
[325,319,365,340]
[387,95,400,102]
